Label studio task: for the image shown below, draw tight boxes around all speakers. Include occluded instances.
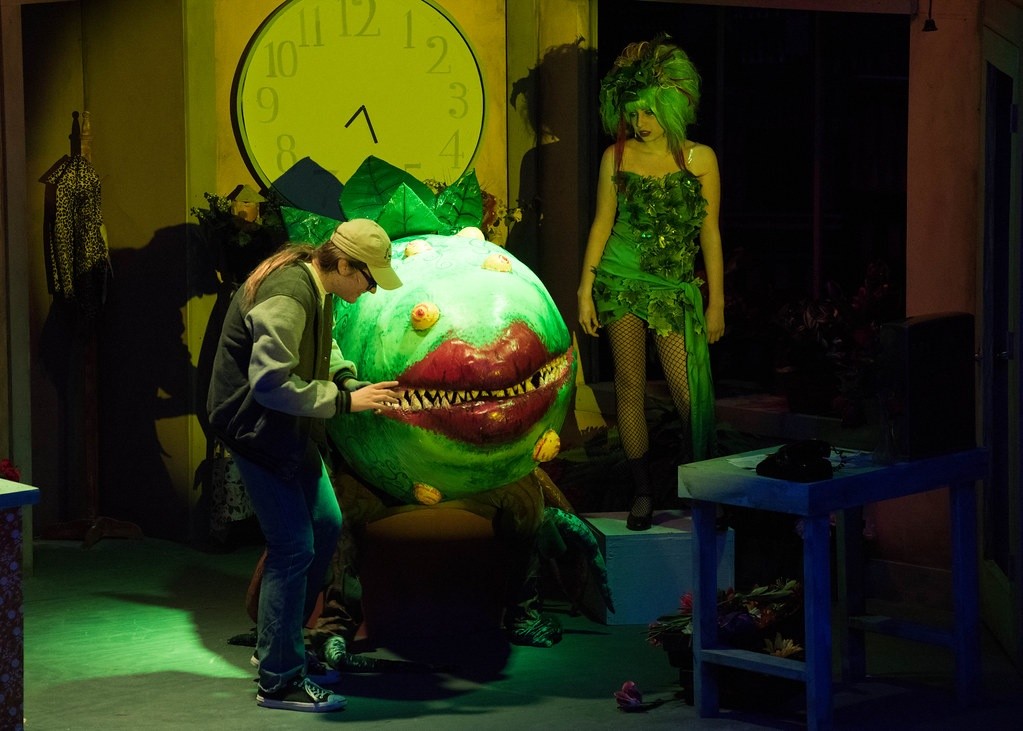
[871,311,974,466]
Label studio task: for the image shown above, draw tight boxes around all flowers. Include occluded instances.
[643,583,805,664]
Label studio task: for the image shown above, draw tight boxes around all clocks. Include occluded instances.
[232,1,487,204]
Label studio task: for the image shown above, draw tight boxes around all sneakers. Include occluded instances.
[256,675,348,713]
[250,649,329,676]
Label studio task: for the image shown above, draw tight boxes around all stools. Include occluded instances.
[581,510,735,628]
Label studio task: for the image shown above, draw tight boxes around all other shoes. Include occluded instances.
[627,495,652,530]
[716,508,728,534]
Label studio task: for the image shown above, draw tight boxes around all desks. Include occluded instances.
[0,478,39,731]
[678,446,1001,731]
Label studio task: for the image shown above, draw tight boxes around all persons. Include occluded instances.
[576,41,725,531]
[207,218,401,714]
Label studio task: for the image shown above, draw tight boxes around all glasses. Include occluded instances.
[351,260,377,291]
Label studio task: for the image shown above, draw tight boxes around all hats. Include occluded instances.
[330,218,402,290]
